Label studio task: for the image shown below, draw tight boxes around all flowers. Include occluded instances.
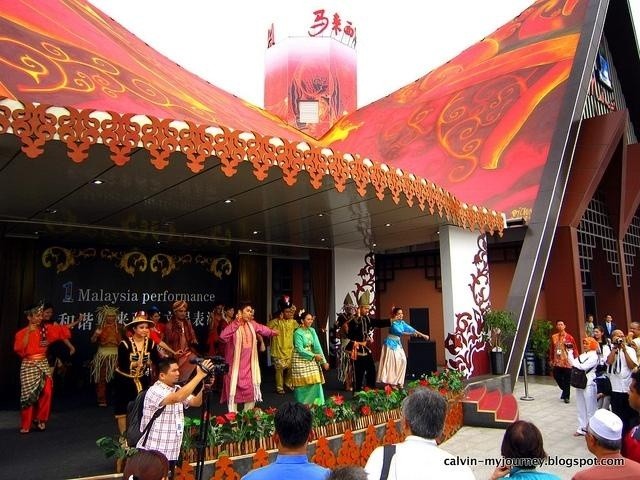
[182,366,466,451]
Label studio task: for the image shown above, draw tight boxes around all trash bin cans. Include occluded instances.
[522,352,535,375]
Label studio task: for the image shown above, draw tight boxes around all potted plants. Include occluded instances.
[529,320,553,376]
[482,305,518,375]
[525,330,536,375]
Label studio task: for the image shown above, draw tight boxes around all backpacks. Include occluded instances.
[569,367,588,389]
[125,386,166,450]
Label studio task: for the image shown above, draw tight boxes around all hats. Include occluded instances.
[589,408,624,441]
[124,310,156,330]
[172,299,189,311]
[356,288,370,308]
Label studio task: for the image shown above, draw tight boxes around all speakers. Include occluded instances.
[409,307,431,341]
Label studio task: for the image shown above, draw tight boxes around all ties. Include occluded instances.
[607,323,611,334]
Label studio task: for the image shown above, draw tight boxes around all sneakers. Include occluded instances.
[560,394,570,404]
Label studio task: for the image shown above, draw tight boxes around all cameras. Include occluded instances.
[564,343,573,348]
[616,338,623,344]
[189,354,230,375]
